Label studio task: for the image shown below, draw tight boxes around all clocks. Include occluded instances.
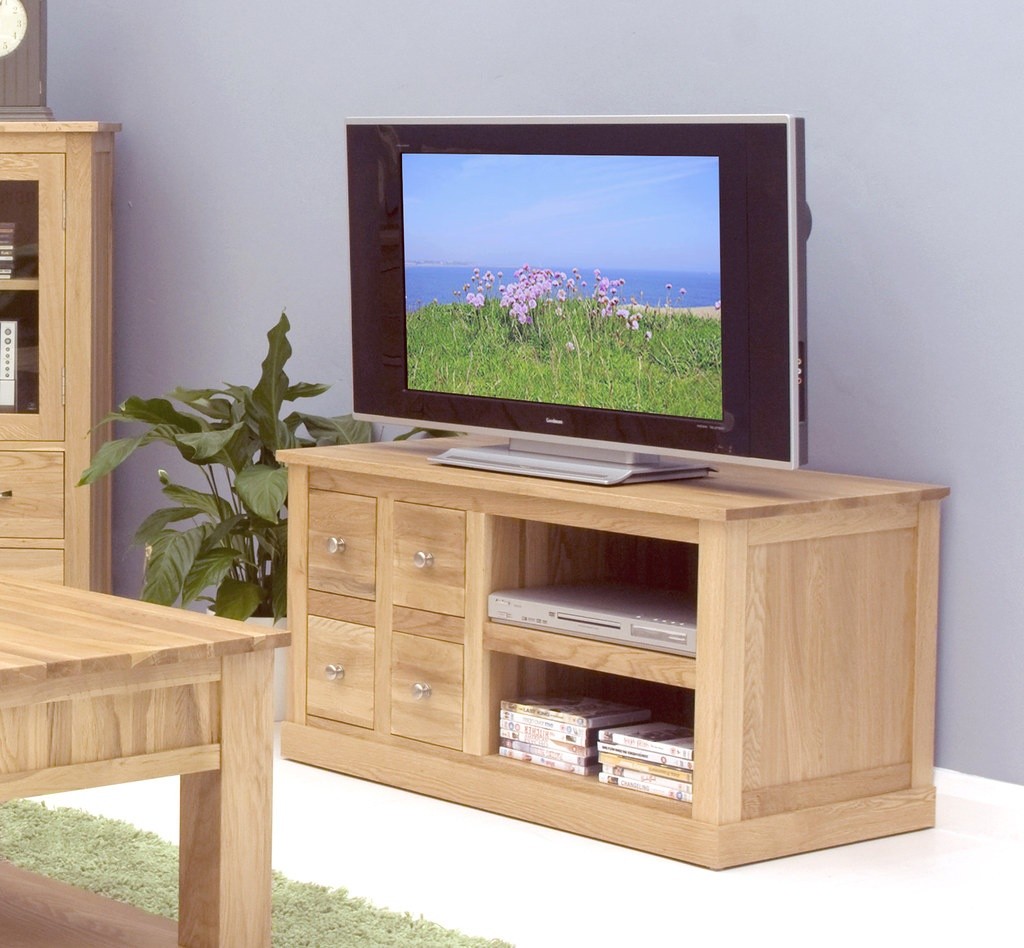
[0,0,52,120]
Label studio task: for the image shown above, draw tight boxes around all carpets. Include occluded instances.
[0,804,517,948]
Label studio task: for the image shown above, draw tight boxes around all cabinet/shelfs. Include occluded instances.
[0,120,121,594]
[275,449,952,871]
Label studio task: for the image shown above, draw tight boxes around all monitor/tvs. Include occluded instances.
[338,115,810,487]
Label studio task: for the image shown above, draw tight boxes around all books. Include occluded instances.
[498,696,694,803]
[0,221,19,280]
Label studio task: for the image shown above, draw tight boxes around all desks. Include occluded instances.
[0,580,293,947]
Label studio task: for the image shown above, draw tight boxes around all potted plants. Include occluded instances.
[75,308,464,726]
